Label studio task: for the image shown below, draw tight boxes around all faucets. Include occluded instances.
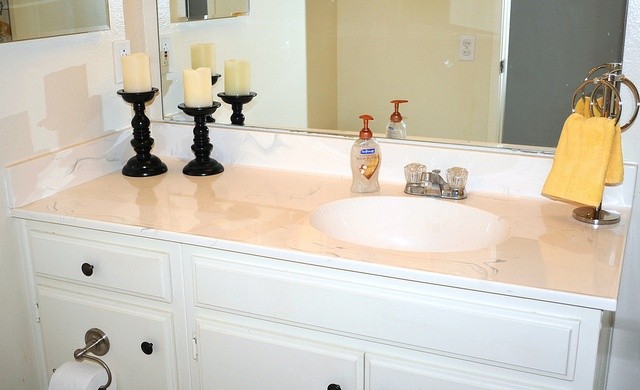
[420,170,448,197]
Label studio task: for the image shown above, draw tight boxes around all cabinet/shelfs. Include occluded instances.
[18,231,171,388]
[185,252,579,390]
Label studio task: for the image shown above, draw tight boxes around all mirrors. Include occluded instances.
[156,1,628,158]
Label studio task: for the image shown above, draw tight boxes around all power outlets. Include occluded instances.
[458,34,475,61]
[159,35,173,74]
[113,41,132,83]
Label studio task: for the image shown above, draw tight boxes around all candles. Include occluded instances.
[188,40,215,75]
[183,65,214,108]
[223,57,250,96]
[120,53,153,93]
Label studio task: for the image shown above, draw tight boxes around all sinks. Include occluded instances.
[310,196,503,253]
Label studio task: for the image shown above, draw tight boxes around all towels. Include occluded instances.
[575,95,605,117]
[542,114,624,207]
